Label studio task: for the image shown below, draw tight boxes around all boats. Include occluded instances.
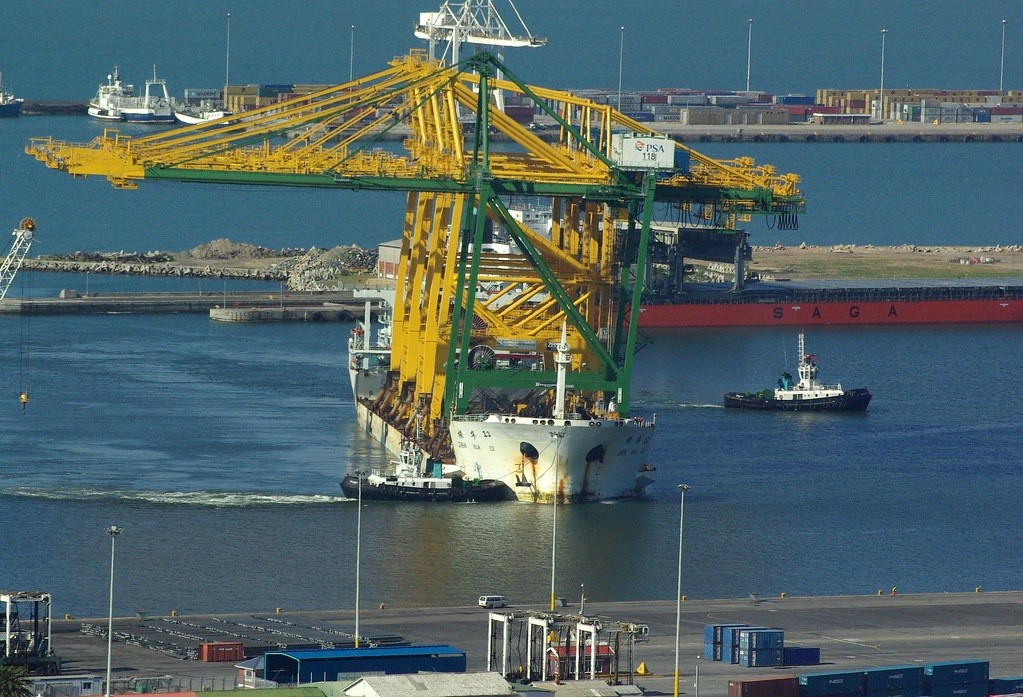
[0,90,24,118]
[25,0,802,506]
[339,410,521,502]
[173,102,244,131]
[88,64,180,124]
[722,331,874,412]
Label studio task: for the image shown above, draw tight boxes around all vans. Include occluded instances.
[479,596,509,608]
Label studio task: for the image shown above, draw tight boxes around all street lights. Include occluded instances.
[1000,19,1009,91]
[674,483,690,696]
[103,527,124,696]
[354,469,369,648]
[617,26,625,113]
[878,28,888,120]
[746,18,755,92]
[549,432,567,612]
[225,13,230,86]
[349,25,355,104]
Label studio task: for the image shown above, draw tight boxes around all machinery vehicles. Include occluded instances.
[0,217,36,416]
